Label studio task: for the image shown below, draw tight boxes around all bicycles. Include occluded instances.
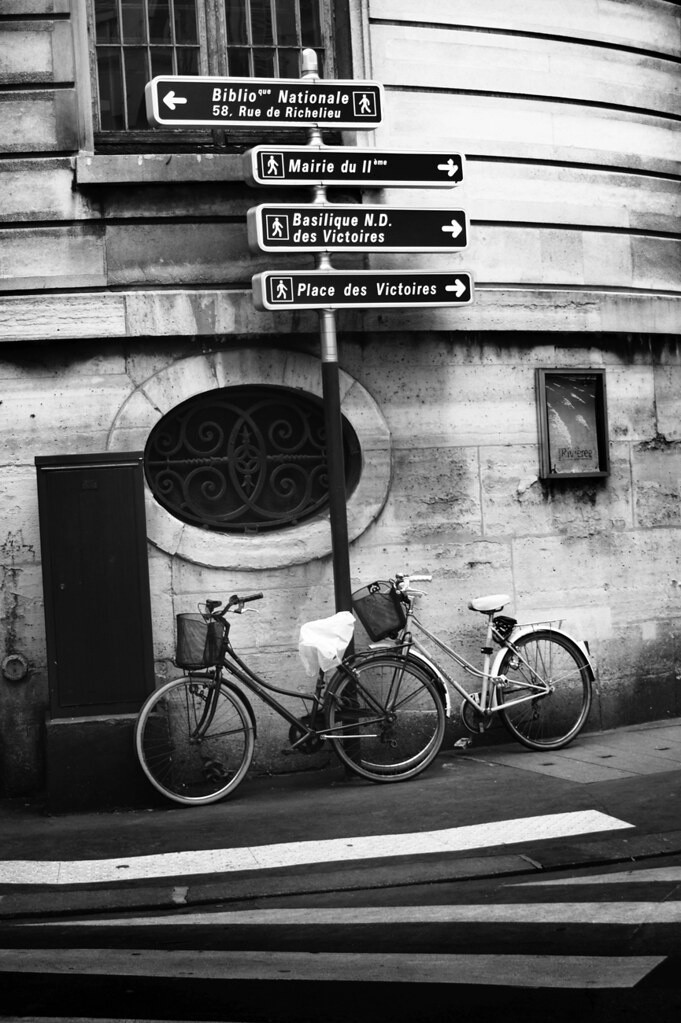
[329,574,596,775]
[129,592,448,804]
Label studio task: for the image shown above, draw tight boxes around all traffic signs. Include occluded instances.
[152,76,384,131]
[247,203,468,253]
[241,144,464,189]
[255,270,473,311]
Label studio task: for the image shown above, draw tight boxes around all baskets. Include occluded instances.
[350,579,407,642]
[175,612,224,669]
